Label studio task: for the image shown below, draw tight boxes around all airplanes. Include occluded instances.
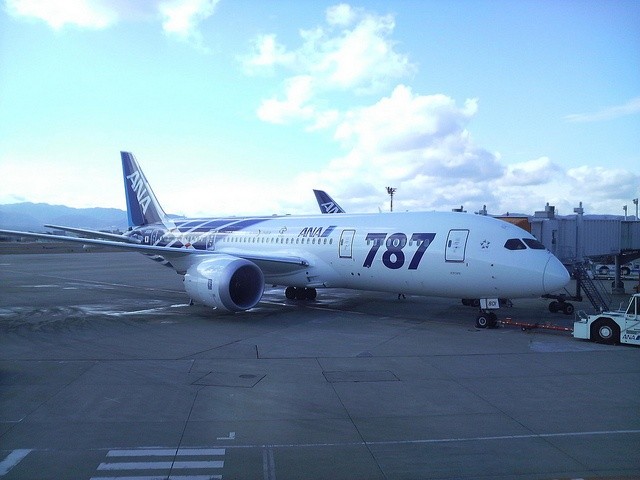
[0,152,571,328]
